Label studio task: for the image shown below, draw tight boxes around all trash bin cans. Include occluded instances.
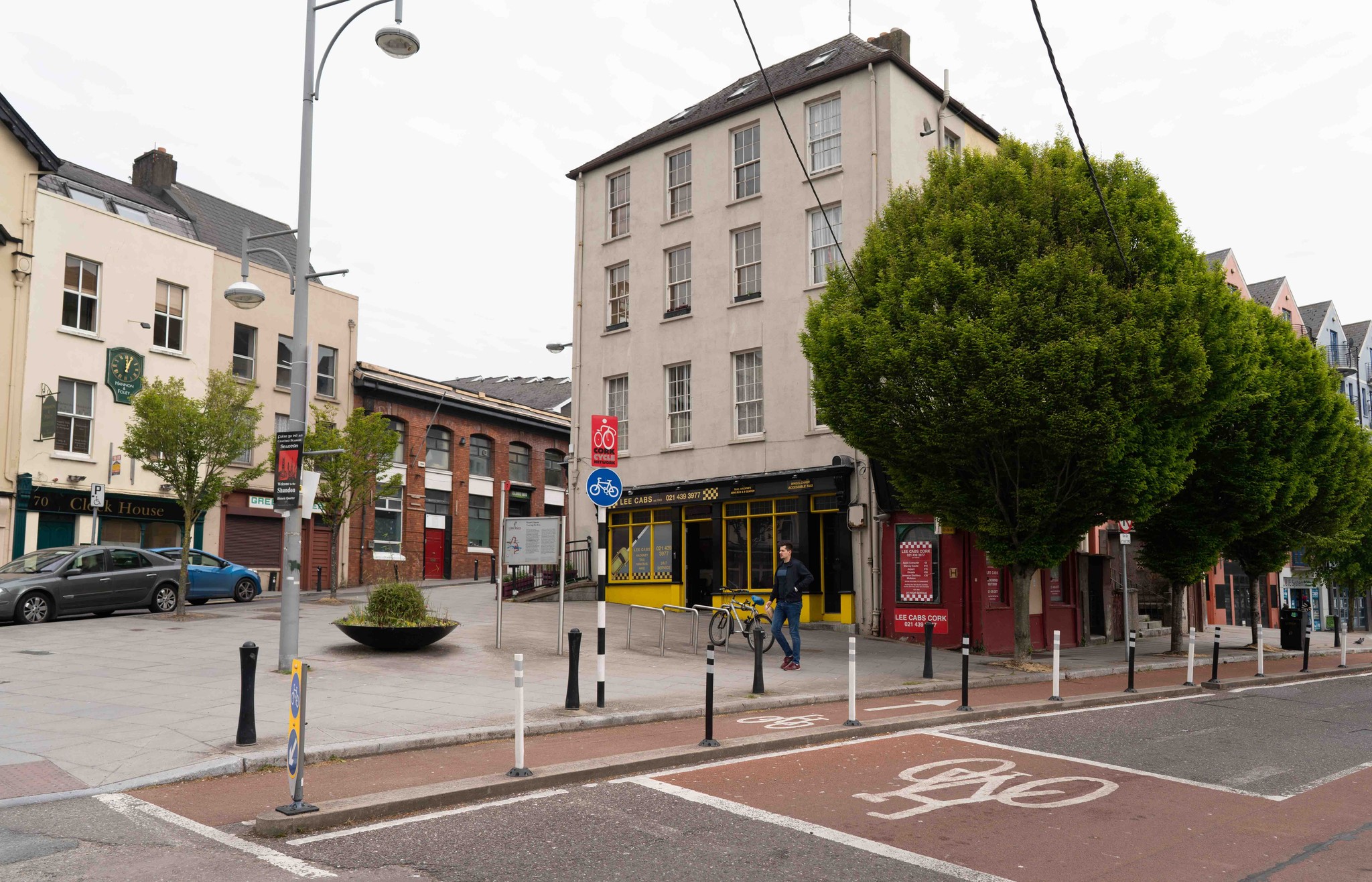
[1280,608,1308,651]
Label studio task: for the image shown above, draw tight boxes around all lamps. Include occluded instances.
[546,342,572,353]
[459,437,466,444]
[1286,295,1290,300]
[128,320,151,329]
[67,475,87,482]
[1231,268,1235,273]
[675,486,681,491]
[160,484,175,491]
[1333,317,1335,321]
[733,480,740,486]
[628,491,634,495]
[792,474,797,479]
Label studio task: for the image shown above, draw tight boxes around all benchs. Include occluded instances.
[114,557,142,569]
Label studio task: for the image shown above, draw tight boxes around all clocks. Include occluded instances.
[111,353,140,382]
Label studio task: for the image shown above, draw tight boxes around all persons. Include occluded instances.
[764,541,814,671]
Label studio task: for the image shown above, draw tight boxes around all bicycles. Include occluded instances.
[708,585,776,653]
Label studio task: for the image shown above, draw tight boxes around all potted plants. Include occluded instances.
[495,569,535,600]
[542,559,579,587]
[328,532,465,651]
[974,638,986,656]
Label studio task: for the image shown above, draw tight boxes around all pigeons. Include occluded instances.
[1352,637,1362,645]
[1354,638,1365,644]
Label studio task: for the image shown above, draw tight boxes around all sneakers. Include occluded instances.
[783,662,800,671]
[781,656,793,668]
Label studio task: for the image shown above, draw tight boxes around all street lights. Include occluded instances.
[222,1,423,675]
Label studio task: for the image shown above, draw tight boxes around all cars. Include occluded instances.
[116,547,263,605]
[0,545,192,625]
